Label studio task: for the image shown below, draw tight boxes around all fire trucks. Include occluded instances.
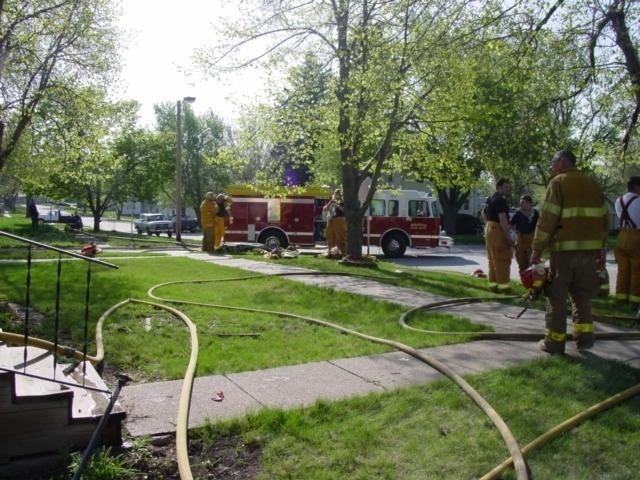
[209,188,455,256]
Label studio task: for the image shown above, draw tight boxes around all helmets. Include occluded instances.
[205,192,224,203]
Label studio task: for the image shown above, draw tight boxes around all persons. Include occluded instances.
[332,188,348,257]
[481,176,516,293]
[63,211,82,232]
[530,149,611,356]
[323,189,341,257]
[509,194,540,288]
[611,174,639,313]
[199,191,220,253]
[29,198,39,231]
[213,193,233,249]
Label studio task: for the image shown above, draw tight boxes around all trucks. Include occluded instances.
[38,209,72,225]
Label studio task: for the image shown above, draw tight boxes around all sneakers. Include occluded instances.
[540,339,593,353]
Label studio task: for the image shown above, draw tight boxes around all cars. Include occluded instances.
[170,213,199,234]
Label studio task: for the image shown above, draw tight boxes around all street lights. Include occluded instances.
[174,95,196,242]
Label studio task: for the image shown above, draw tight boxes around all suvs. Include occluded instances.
[132,211,174,239]
